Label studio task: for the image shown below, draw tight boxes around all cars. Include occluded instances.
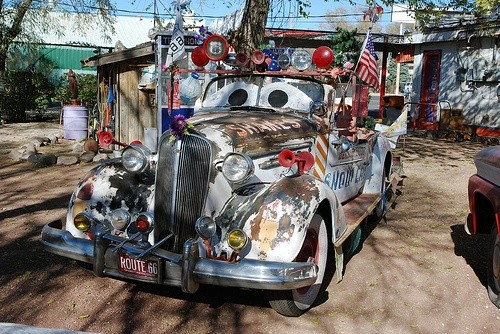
[38,67,404,319]
[464,144,500,308]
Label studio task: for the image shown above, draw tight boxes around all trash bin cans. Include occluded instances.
[380,95,405,126]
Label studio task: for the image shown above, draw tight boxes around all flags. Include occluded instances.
[355,30,380,94]
[163,11,185,71]
